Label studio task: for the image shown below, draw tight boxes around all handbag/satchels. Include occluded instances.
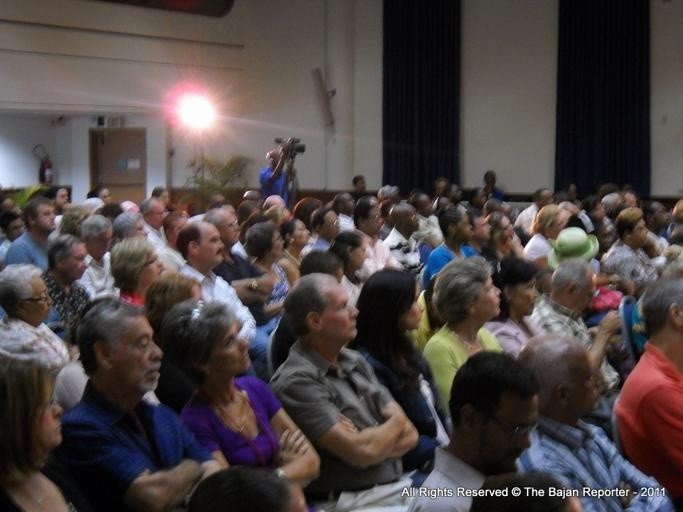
[587,289,625,312]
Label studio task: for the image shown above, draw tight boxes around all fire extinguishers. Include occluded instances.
[43,159,52,186]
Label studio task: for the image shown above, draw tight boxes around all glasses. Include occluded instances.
[143,254,158,266]
[17,290,50,302]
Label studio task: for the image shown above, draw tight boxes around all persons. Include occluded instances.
[0,169,683,512]
[259,144,296,208]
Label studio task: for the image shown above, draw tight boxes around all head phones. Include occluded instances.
[265,150,274,162]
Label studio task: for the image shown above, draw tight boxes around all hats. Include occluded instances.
[546,226,600,270]
